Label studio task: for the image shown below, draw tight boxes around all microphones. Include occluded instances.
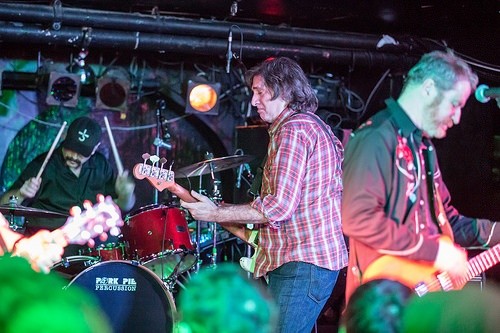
[225,31,233,73]
[236,163,243,188]
[474,84,500,103]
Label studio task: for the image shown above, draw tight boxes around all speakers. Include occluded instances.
[232,124,273,206]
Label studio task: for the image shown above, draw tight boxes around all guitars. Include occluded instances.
[10,193,125,273]
[132,152,259,274]
[362,236,500,296]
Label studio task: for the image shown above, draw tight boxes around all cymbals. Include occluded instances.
[0,205,69,218]
[174,154,256,178]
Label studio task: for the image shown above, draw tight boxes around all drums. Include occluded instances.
[48,255,178,333]
[187,219,232,249]
[78,242,124,262]
[121,199,199,283]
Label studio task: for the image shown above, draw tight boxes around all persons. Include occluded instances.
[0,250,39,275]
[341,51,500,328]
[0,117,136,279]
[181,57,347,333]
[175,263,279,333]
[0,211,64,273]
[0,275,112,333]
[403,289,500,333]
[344,279,411,333]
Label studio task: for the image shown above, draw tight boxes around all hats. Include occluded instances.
[60,117,103,158]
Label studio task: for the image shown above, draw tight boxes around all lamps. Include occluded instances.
[179,70,221,116]
[36,64,82,108]
[95,65,131,113]
